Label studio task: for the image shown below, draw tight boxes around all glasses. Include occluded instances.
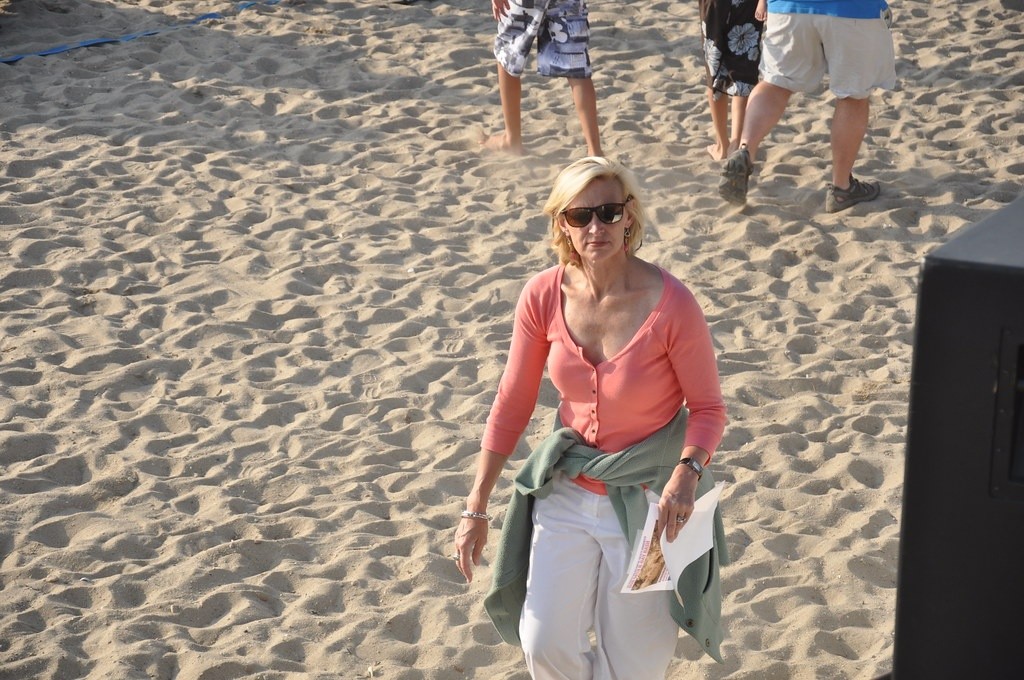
[560,194,633,228]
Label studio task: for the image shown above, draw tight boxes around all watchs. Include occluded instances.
[676,456,704,482]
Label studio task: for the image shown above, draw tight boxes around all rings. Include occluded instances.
[453,554,460,560]
[677,517,686,523]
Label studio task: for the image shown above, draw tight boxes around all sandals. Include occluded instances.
[825,172,880,213]
[719,143,754,205]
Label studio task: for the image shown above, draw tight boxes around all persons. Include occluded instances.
[699,0,765,161]
[453,155,727,679]
[717,0,897,214]
[483,0,603,157]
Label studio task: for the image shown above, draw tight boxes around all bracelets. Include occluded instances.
[459,511,492,520]
